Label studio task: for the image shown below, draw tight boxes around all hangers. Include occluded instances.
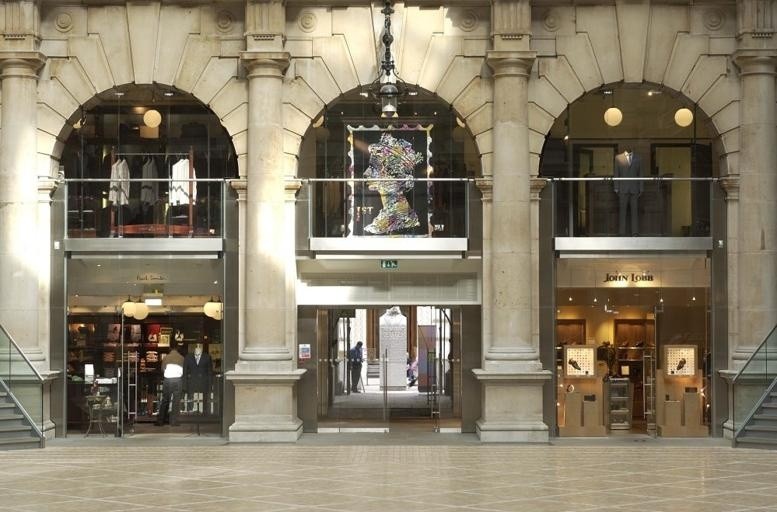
[172,152,190,168]
[142,153,157,168]
[111,152,129,169]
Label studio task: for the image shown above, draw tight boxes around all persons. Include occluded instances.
[613,146,644,237]
[349,341,364,392]
[154,341,185,426]
[186,341,214,411]
[362,133,423,235]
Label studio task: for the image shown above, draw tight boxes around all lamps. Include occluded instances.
[604,89,623,127]
[122,296,149,320]
[143,86,162,128]
[367,0,409,118]
[674,105,693,127]
[203,296,223,321]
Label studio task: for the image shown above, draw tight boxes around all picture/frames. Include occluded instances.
[342,118,437,238]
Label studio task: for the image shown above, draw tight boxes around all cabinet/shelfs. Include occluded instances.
[614,319,655,420]
[83,395,108,438]
[556,319,586,368]
[68,316,222,434]
[609,378,630,430]
[585,174,671,236]
